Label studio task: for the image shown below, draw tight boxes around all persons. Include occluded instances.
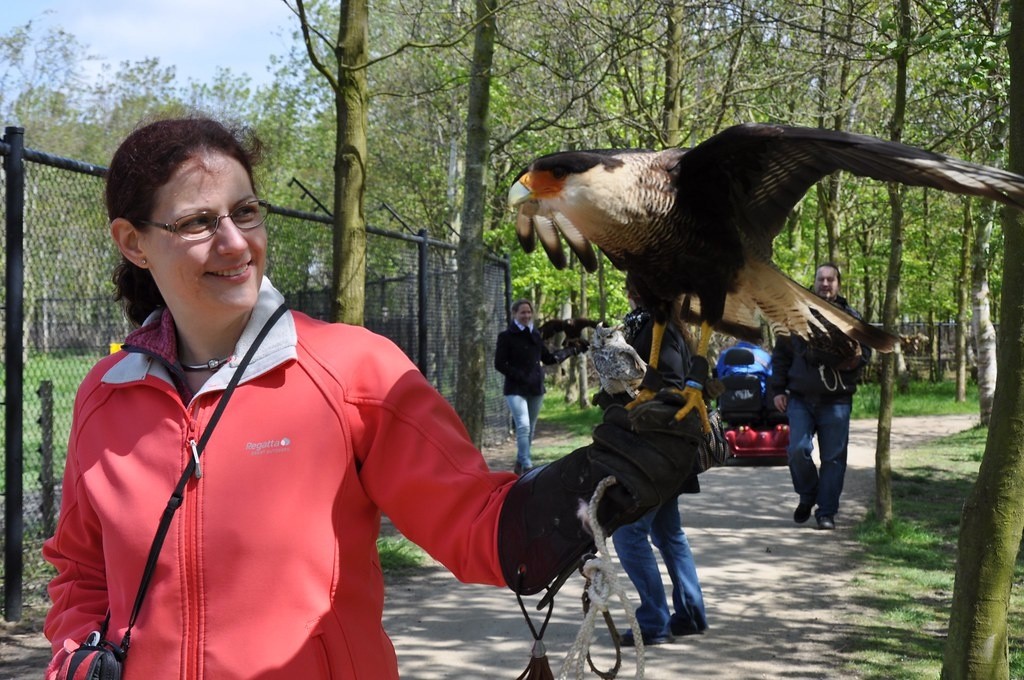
[494,301,579,476]
[596,273,707,644]
[771,263,871,530]
[717,332,774,397]
[43,120,718,679]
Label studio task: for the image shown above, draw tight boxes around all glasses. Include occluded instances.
[132,199,271,241]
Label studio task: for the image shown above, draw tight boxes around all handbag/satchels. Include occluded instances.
[695,406,729,474]
[55,631,126,680]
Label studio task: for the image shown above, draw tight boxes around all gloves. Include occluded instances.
[498,396,701,595]
[552,345,584,364]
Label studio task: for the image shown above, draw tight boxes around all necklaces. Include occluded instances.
[181,356,232,372]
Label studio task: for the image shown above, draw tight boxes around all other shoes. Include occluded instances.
[794,502,816,523]
[671,613,706,635]
[618,631,675,646]
[817,515,834,529]
[514,463,532,475]
